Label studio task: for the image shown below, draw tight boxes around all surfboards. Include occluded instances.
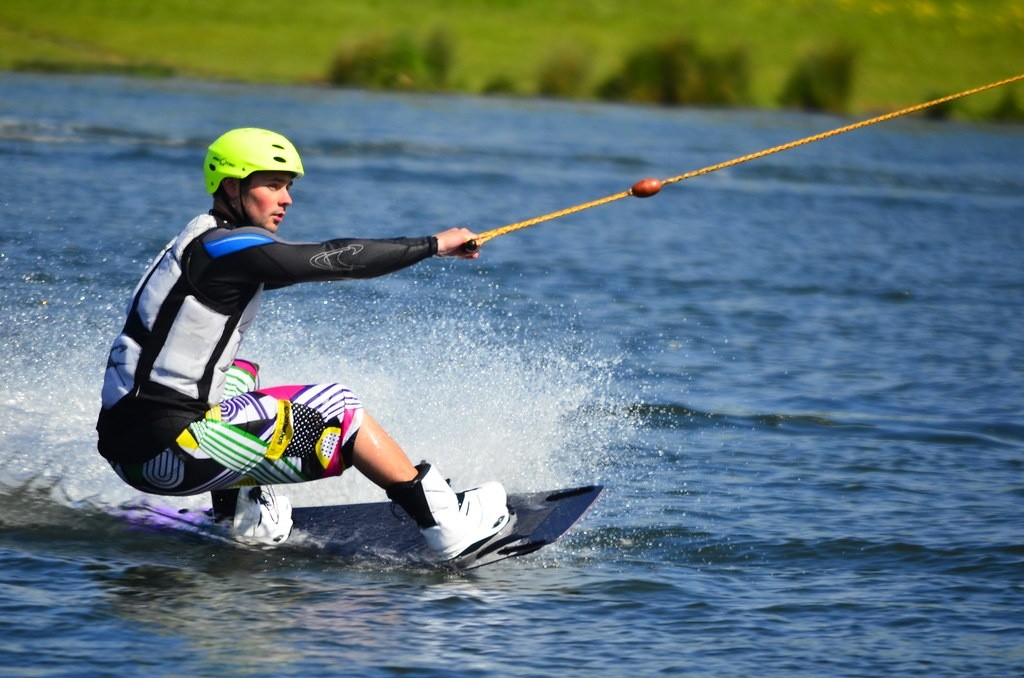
[120,485,603,571]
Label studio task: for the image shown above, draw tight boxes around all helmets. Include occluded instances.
[203,126,306,195]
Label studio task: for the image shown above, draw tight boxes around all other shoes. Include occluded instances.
[210,485,293,545]
[385,459,510,562]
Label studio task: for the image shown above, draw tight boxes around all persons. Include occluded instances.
[95,126,508,561]
